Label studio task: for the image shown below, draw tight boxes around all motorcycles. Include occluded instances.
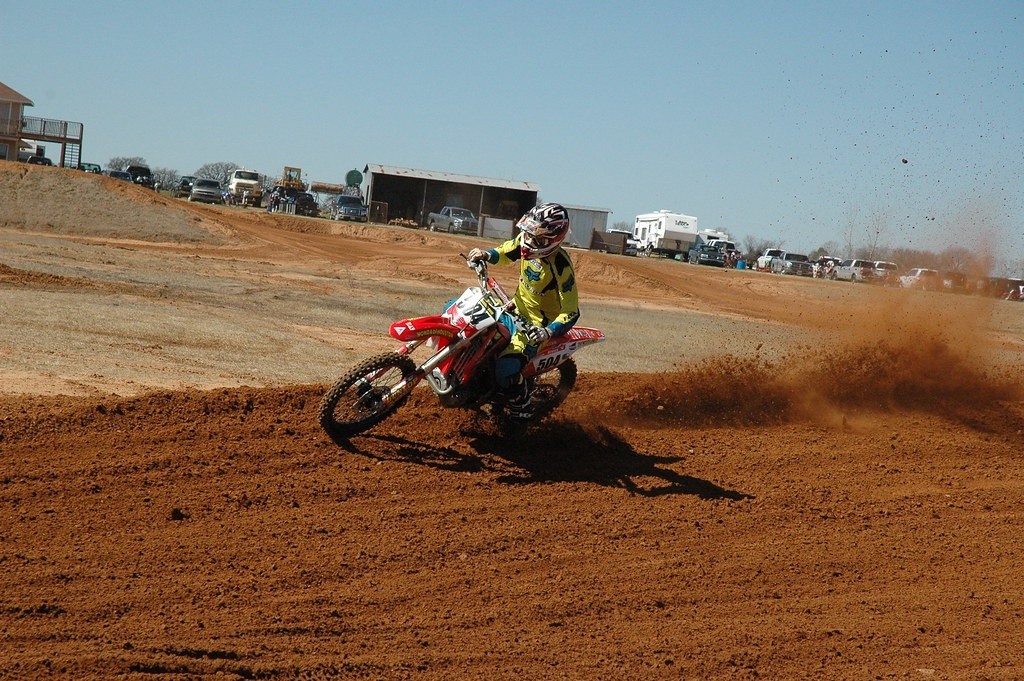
[318,251,605,436]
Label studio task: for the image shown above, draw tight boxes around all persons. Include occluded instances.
[469,203,580,439]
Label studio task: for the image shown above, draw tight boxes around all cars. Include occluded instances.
[943,272,1024,301]
[285,193,321,217]
[188,178,222,205]
[26,156,52,166]
[77,164,102,175]
[106,170,133,183]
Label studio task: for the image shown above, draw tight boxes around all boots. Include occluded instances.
[510,379,536,421]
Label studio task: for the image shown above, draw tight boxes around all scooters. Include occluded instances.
[817,265,825,278]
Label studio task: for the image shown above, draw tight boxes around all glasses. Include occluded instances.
[524,230,549,248]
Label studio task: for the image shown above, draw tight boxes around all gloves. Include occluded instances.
[466,247,491,264]
[529,321,554,343]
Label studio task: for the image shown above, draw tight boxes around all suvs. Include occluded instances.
[606,229,637,256]
[812,253,842,277]
[898,267,940,292]
[832,260,877,282]
[329,196,369,223]
[706,240,736,255]
[124,165,154,188]
[871,261,899,286]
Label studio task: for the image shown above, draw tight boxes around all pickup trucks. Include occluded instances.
[770,253,815,277]
[427,206,478,235]
[756,248,786,268]
[173,176,197,198]
[688,245,723,267]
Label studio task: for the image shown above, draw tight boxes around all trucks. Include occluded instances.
[227,168,263,207]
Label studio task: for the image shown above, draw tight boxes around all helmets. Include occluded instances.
[515,203,570,260]
